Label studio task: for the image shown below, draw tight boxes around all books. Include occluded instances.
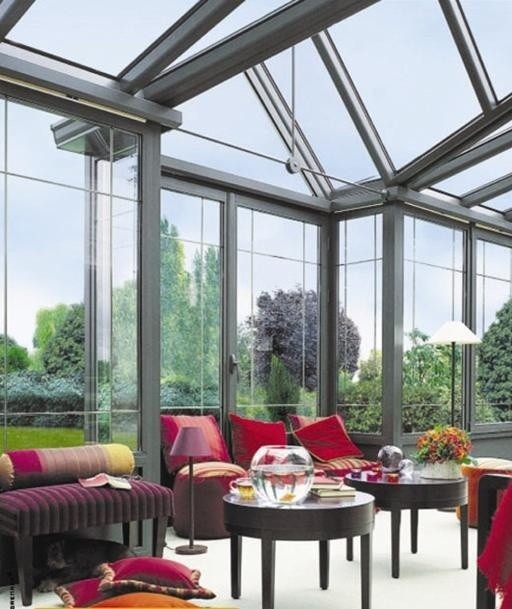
[77,472,132,491]
[309,475,356,499]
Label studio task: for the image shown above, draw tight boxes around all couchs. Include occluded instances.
[0,444,175,607]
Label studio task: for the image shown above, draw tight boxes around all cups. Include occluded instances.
[230,477,254,499]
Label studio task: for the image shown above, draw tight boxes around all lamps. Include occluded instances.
[170,426,211,554]
[427,321,482,426]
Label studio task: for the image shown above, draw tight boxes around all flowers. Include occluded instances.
[410,423,477,467]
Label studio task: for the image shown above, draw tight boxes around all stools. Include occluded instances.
[455,457,512,528]
[171,461,249,540]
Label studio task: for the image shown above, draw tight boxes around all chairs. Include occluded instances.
[477,474,511,609]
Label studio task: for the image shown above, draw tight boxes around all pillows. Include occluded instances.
[314,459,380,475]
[227,412,287,469]
[287,414,329,431]
[160,414,232,472]
[88,592,200,609]
[54,577,110,609]
[0,442,136,490]
[98,557,217,600]
[294,416,364,462]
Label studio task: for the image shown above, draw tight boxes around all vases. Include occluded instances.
[420,458,462,479]
